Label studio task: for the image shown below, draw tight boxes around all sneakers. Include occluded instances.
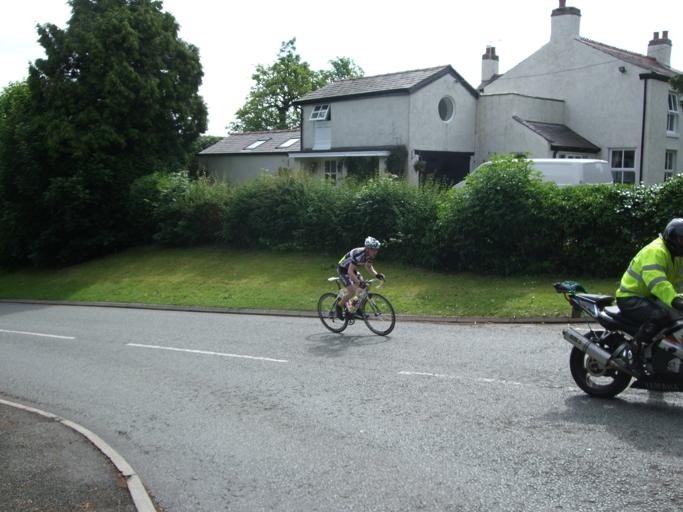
[335,303,344,320]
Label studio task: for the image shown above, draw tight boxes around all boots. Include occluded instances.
[624,347,651,378]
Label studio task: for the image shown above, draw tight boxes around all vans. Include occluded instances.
[451,158,614,198]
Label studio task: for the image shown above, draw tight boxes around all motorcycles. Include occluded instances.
[553,280,683,398]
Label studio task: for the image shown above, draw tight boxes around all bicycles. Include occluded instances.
[317,273,396,336]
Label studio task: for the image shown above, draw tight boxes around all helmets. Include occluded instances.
[365,236,380,249]
[664,218,683,256]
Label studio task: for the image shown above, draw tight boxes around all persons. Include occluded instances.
[335,236,384,321]
[615,217,683,381]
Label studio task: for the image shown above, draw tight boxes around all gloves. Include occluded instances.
[672,297,683,309]
[359,281,366,289]
[376,273,384,280]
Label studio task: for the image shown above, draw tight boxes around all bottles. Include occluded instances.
[352,297,358,307]
